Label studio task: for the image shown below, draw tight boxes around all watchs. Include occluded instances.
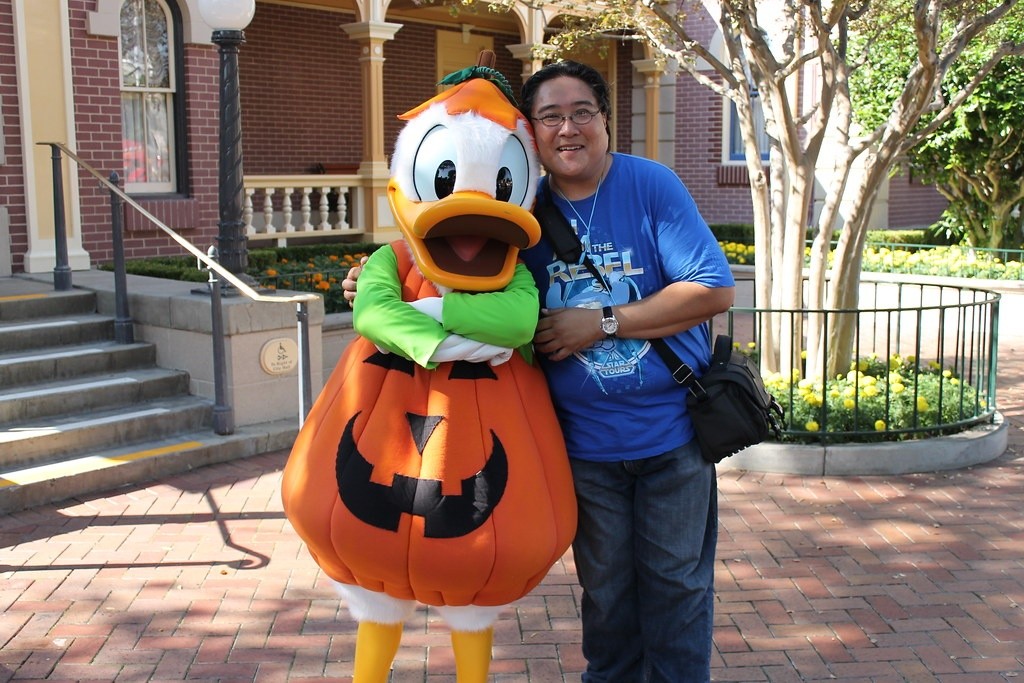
[600,306,620,339]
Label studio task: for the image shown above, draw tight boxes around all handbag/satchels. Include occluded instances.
[686,335,788,464]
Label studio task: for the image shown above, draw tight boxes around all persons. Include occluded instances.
[342,60,735,683]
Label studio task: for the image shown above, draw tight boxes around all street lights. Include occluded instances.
[190,0,276,296]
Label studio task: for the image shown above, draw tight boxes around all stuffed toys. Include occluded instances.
[282,51,577,683]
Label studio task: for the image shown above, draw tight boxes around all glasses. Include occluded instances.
[530,107,603,127]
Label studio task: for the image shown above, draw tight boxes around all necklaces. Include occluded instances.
[552,176,617,306]
